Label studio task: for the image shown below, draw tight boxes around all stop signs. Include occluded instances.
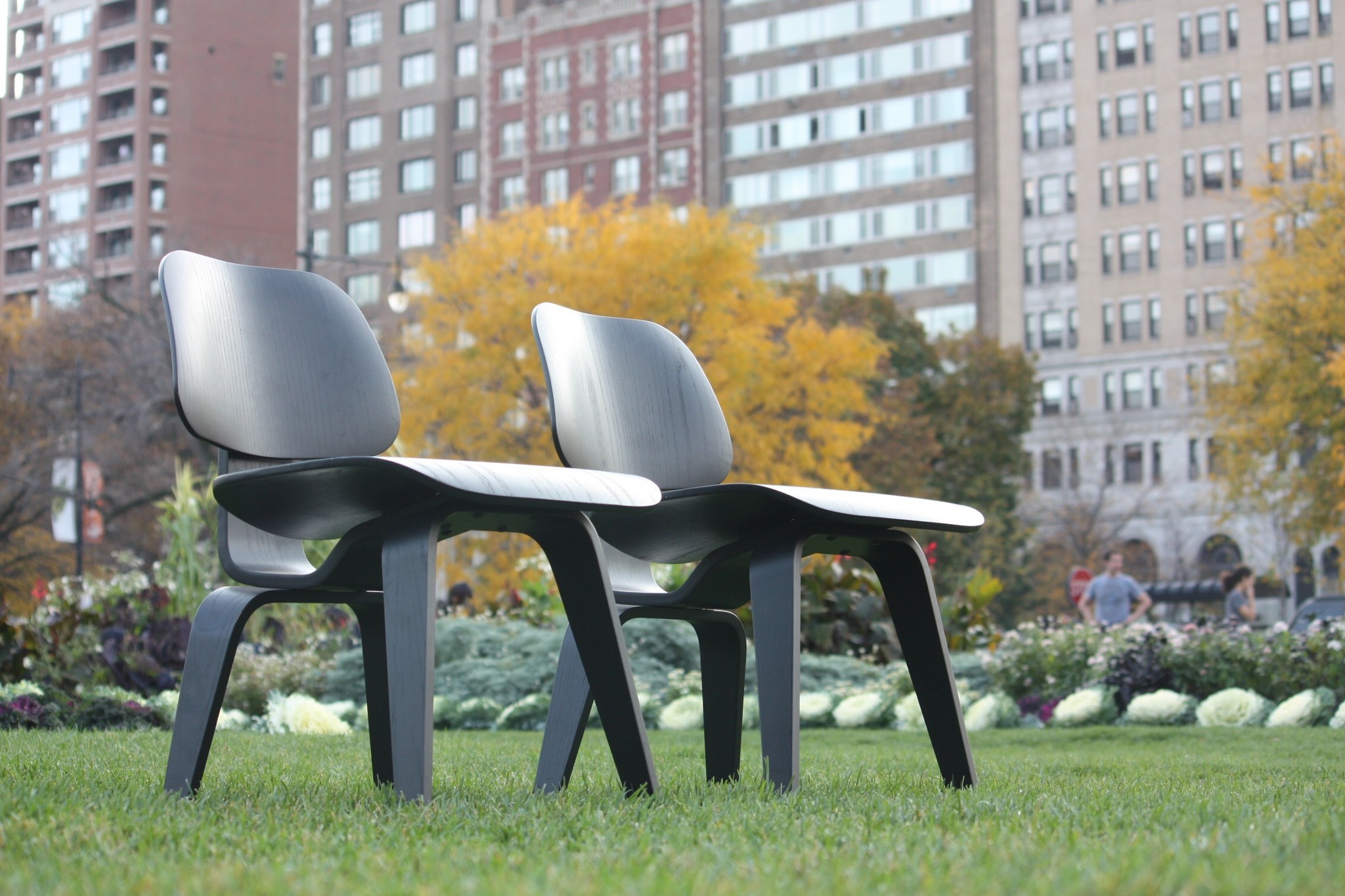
[1071,570,1092,603]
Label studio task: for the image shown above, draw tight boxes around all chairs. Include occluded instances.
[160,249,661,802]
[533,299,987,797]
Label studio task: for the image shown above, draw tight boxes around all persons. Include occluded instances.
[1078,549,1153,633]
[1223,565,1257,627]
